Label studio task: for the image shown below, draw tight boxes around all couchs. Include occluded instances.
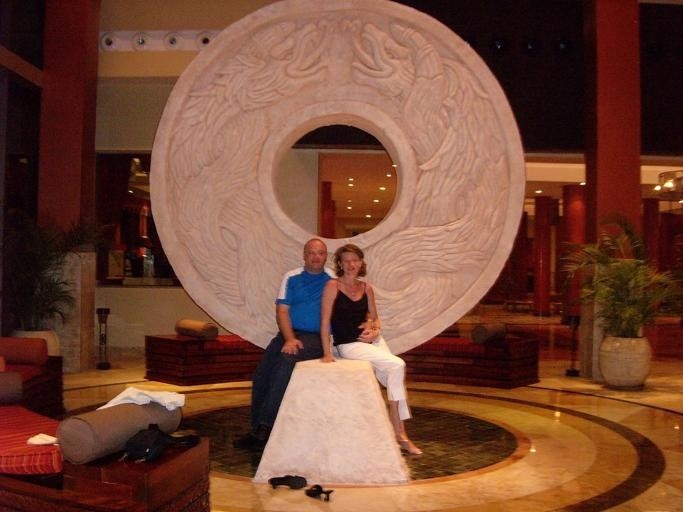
[1,338,210,512]
[144,319,541,385]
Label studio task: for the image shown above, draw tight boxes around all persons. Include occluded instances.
[319,243,423,455]
[231,238,373,449]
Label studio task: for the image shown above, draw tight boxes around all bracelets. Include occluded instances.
[373,326,380,329]
[368,319,375,324]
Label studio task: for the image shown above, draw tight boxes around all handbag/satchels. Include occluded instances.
[118,424,197,463]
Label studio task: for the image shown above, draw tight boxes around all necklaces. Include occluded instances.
[342,278,360,298]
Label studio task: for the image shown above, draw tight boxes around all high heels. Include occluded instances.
[306,484,333,501]
[268,475,305,489]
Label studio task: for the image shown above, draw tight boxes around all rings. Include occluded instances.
[367,340,372,343]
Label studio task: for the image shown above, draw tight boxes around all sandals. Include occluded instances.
[398,436,422,455]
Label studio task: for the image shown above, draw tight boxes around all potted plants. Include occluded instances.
[0,199,98,356]
[553,214,683,390]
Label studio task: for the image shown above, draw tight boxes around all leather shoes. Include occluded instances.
[234,433,266,449]
